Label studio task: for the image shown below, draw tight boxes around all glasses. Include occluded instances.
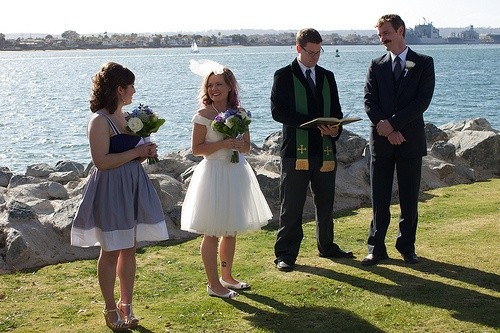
[301,46,325,57]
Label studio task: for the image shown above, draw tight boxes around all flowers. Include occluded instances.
[124,104,165,165]
[211,107,251,163]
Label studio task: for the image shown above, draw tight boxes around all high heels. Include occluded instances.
[103,304,129,332]
[116,297,139,329]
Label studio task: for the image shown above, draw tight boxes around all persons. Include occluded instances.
[71,61,169,333]
[270,28,353,270]
[180,60,273,297]
[361,14,435,267]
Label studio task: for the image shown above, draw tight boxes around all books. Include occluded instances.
[299,117,363,130]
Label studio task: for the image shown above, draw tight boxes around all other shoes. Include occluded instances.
[220,275,251,289]
[207,286,240,298]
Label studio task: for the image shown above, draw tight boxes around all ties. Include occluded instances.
[393,56,402,92]
[305,69,316,101]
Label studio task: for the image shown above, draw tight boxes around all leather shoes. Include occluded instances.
[360,252,389,267]
[395,244,420,264]
[277,261,293,271]
[319,247,353,258]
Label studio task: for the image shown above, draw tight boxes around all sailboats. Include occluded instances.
[190,39,199,53]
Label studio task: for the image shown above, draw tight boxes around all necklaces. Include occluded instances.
[212,102,218,113]
